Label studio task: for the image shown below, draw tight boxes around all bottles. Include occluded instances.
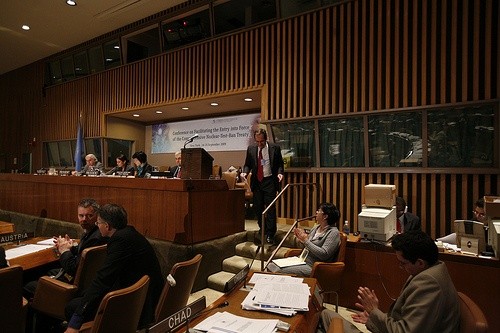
[342,220,350,241]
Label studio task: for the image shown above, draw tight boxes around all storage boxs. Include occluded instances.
[484,196,500,217]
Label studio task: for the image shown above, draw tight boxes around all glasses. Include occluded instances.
[397,209,402,212]
[399,260,408,266]
[472,211,484,217]
[94,221,103,225]
[316,210,324,214]
[86,160,91,162]
[117,161,121,163]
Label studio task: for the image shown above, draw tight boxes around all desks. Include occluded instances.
[0,237,82,272]
[173,270,324,333]
[0,173,247,245]
[323,233,500,333]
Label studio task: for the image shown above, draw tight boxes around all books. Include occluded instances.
[192,272,312,333]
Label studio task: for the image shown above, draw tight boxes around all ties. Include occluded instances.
[174,167,179,177]
[396,218,401,233]
[256,148,263,182]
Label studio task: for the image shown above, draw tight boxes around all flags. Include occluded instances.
[73,125,83,172]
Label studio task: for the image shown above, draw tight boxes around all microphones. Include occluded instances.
[243,246,261,288]
[186,302,229,333]
[187,134,198,140]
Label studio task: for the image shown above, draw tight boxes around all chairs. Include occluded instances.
[0,265,25,333]
[155,253,203,324]
[159,166,169,172]
[32,244,108,318]
[89,275,150,333]
[457,291,489,332]
[222,172,236,189]
[284,233,347,299]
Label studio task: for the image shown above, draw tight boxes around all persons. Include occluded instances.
[169,151,183,178]
[472,200,494,254]
[153,125,170,152]
[64,204,166,333]
[83,154,105,174]
[267,202,341,277]
[129,151,152,177]
[350,228,461,333]
[240,124,284,242]
[115,155,134,176]
[395,196,421,233]
[25,199,107,295]
